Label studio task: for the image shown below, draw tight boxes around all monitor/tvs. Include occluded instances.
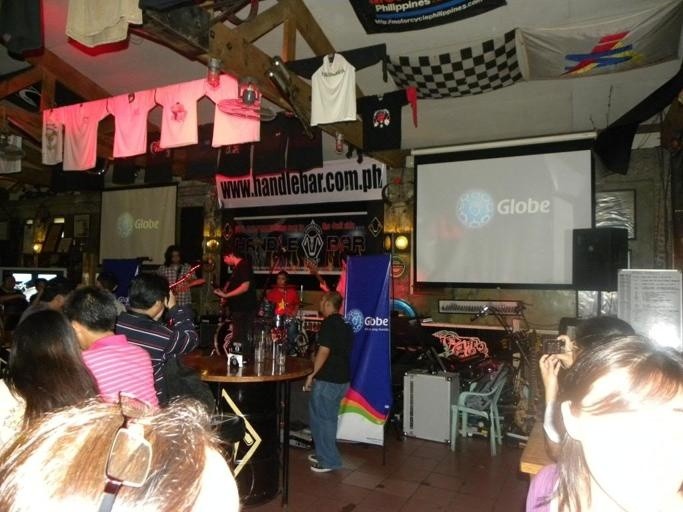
[12,274,32,293]
[38,271,58,282]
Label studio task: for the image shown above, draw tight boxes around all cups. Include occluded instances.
[274,342,286,366]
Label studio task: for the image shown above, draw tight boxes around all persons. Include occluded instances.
[212,242,257,352]
[156,244,197,309]
[0,271,200,465]
[268,271,300,320]
[303,288,355,474]
[523,312,683,512]
[0,392,239,512]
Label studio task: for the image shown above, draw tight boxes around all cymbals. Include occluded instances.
[286,300,314,307]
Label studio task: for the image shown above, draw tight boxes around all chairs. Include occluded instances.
[449,367,510,456]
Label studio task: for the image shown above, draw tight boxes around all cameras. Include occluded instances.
[542,339,565,354]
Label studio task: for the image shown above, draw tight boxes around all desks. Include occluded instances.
[179,350,316,508]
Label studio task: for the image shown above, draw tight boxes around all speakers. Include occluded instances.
[573,228,628,291]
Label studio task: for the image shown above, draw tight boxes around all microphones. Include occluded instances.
[137,257,152,261]
[471,307,489,321]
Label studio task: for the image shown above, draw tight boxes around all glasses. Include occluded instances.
[100,391,153,512]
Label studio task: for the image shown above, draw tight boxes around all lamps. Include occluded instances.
[208,58,220,86]
[206,239,220,250]
[383,231,411,254]
[241,76,257,105]
[335,132,344,152]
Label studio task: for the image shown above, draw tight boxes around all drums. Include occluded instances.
[273,313,300,337]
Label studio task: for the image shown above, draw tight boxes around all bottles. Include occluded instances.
[250,303,266,363]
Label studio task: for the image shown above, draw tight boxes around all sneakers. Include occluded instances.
[308,454,332,472]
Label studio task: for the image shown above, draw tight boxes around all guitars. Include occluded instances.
[169,264,200,288]
[215,280,229,304]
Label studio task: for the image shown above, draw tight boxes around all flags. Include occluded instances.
[347,0,683,101]
